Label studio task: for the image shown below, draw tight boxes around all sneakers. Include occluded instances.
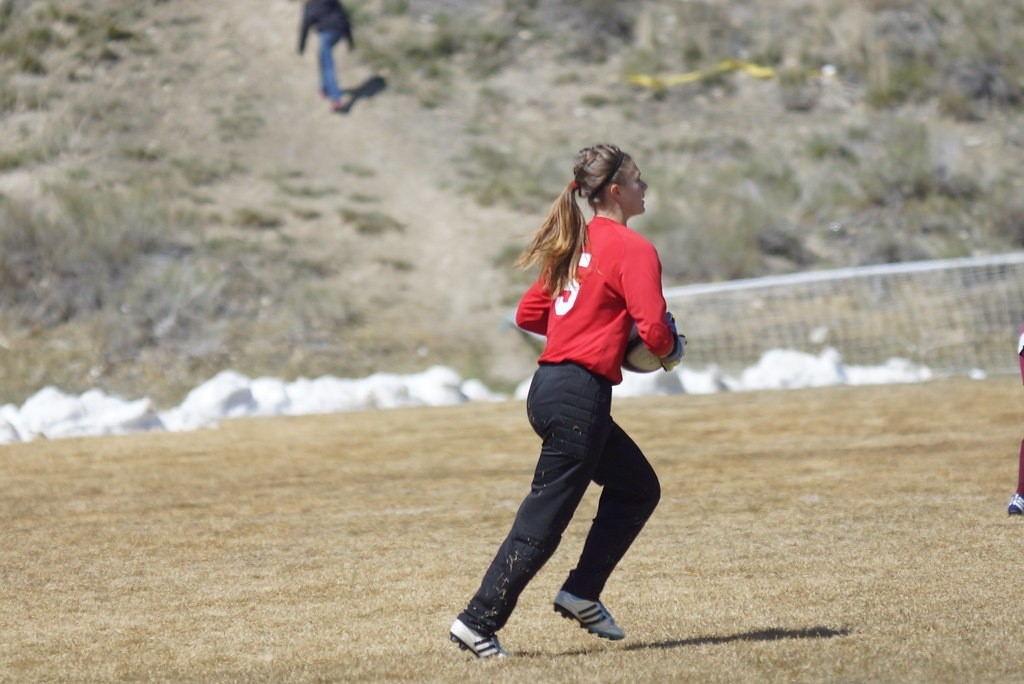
[449,618,512,659]
[1008,492,1024,515]
[554,590,626,640]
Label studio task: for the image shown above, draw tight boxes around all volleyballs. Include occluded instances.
[621,323,667,374]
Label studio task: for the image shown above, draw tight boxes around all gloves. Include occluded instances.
[661,312,688,371]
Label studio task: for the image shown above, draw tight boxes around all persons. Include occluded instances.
[1006,313,1023,518]
[295,0,362,113]
[447,141,688,662]
